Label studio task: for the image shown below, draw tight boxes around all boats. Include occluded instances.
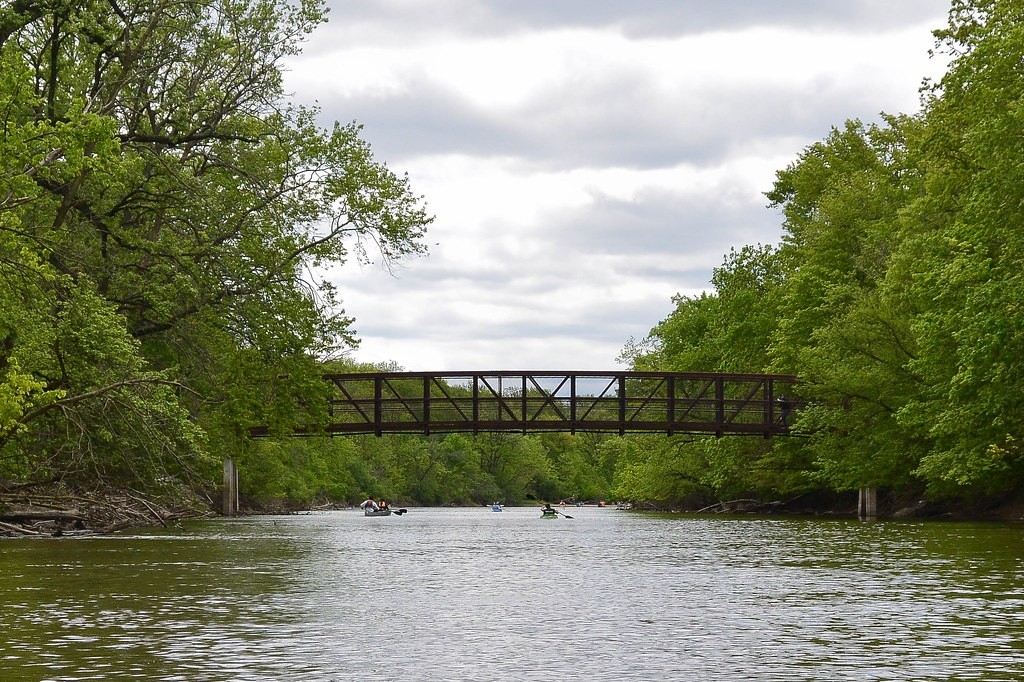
[491,505,503,512]
[364,504,392,517]
[540,513,559,520]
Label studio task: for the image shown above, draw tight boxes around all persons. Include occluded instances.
[541,503,558,515]
[492,501,502,510]
[360,496,388,512]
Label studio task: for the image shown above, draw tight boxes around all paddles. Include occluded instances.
[553,509,576,520]
[383,510,403,516]
[388,507,408,513]
